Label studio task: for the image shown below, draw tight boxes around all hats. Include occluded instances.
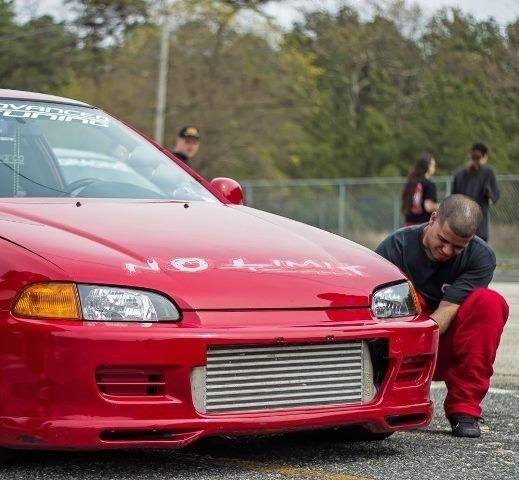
[178,126,202,139]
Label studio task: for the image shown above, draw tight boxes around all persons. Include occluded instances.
[173,125,201,166]
[402,142,500,243]
[374,194,509,437]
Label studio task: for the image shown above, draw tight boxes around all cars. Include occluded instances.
[0,86,440,450]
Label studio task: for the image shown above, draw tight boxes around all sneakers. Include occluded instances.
[448,413,481,438]
